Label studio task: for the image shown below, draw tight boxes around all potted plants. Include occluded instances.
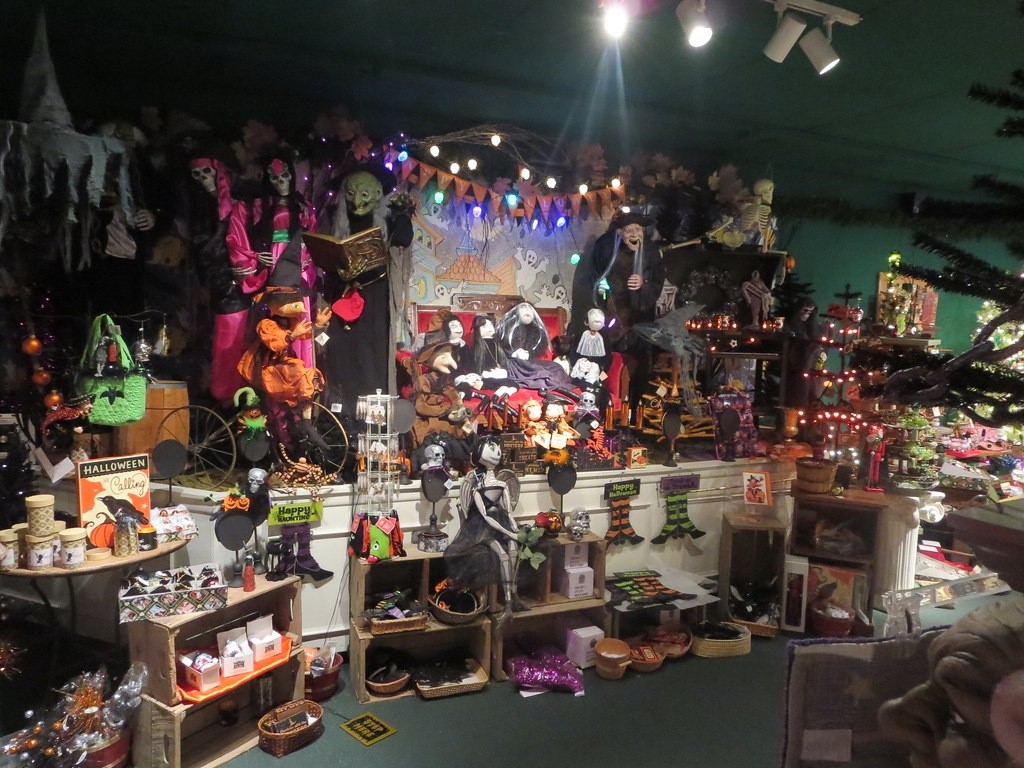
[791,422,837,495]
[883,403,940,491]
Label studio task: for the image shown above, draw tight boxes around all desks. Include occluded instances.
[0,537,193,686]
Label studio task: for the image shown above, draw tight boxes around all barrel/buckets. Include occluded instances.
[595,638,631,680]
[797,456,838,493]
[304,652,343,703]
[808,599,856,638]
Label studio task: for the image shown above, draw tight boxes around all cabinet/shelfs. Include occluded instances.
[782,492,929,640]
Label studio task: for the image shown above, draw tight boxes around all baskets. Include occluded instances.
[594,599,780,680]
[795,457,838,494]
[257,699,326,758]
[370,614,428,634]
[415,658,489,699]
[365,663,412,694]
[428,586,487,625]
[304,652,344,702]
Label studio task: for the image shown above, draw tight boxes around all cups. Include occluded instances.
[138,528,158,550]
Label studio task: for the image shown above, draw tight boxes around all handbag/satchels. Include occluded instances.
[70,313,149,426]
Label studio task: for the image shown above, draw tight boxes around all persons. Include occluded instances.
[178,147,415,483]
[423,300,580,413]
[740,178,775,235]
[458,434,533,629]
[784,295,824,412]
[591,212,664,425]
[71,106,181,347]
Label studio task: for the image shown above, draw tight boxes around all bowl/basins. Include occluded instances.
[630,644,666,672]
[428,598,485,624]
[365,673,411,693]
[651,624,692,658]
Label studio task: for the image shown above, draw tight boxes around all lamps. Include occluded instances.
[762,1,807,64]
[799,15,840,76]
[675,0,713,47]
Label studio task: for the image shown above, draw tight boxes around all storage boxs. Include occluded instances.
[555,567,594,599]
[181,649,220,693]
[715,513,786,638]
[131,648,305,768]
[555,543,588,569]
[522,529,608,602]
[350,618,489,705]
[117,561,228,624]
[247,613,282,662]
[490,598,612,682]
[568,625,605,668]
[217,627,254,677]
[129,573,301,707]
[350,540,489,631]
[783,555,808,633]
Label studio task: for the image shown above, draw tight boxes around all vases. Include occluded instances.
[813,603,857,640]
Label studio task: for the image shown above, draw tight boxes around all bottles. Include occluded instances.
[114,517,139,557]
[52,520,66,560]
[26,494,55,537]
[11,523,29,564]
[24,533,55,570]
[252,672,275,709]
[0,529,19,571]
[243,556,255,592]
[59,528,87,568]
